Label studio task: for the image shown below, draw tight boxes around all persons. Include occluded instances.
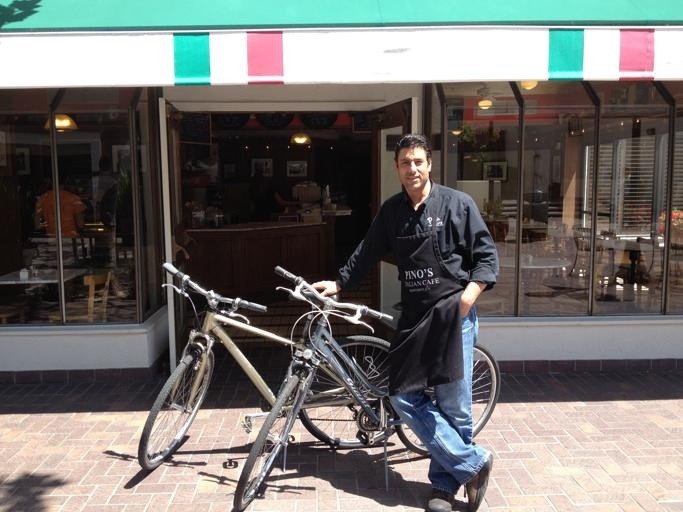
[25,154,134,268]
[249,161,301,222]
[302,133,500,512]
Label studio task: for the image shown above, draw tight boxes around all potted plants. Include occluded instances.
[480,199,504,218]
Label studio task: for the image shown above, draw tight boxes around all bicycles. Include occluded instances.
[137,261,397,467]
[230,265,501,512]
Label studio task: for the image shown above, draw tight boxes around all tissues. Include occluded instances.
[20,267,30,281]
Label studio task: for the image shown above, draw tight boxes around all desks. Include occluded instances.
[483,217,513,243]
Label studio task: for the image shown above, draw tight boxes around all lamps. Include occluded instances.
[294,128,307,144]
[477,87,495,110]
[45,113,78,132]
[245,113,262,129]
[521,82,538,90]
[333,111,355,128]
[289,112,304,127]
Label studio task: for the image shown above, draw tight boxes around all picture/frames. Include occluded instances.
[251,159,272,177]
[481,161,508,182]
[286,160,307,177]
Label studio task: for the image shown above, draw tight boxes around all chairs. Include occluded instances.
[0,305,27,324]
[569,227,683,302]
[48,271,112,324]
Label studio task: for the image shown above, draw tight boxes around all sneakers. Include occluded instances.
[464,462,489,512]
[426,488,455,512]
